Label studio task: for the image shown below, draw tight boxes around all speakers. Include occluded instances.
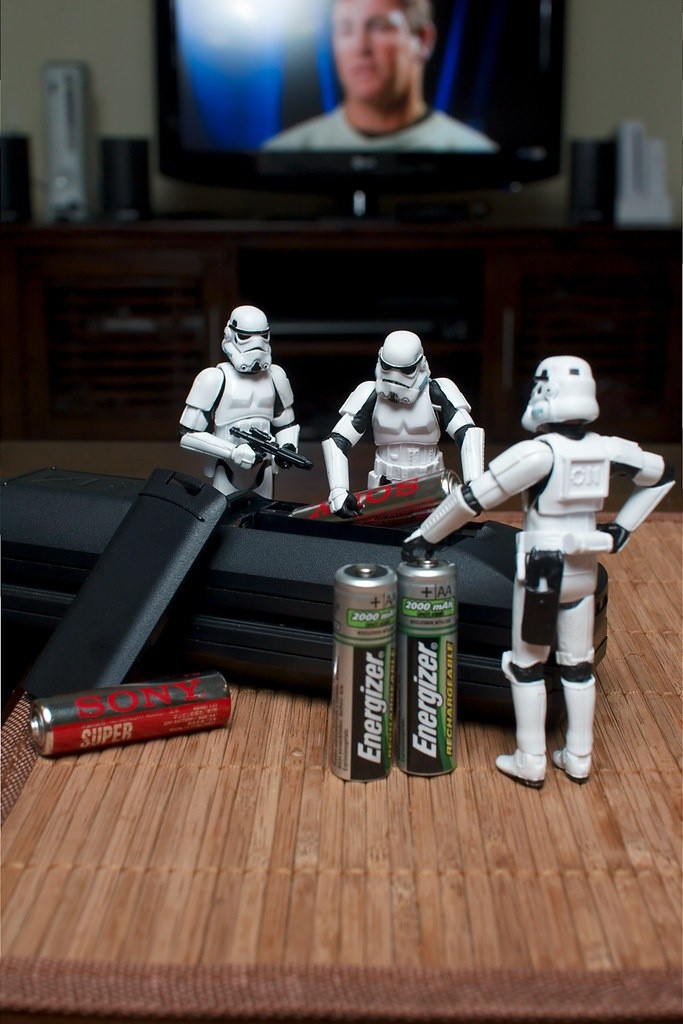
[569,140,617,226]
[102,137,149,225]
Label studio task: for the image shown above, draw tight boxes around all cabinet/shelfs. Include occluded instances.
[1,209,682,446]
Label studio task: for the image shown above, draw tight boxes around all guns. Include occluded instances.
[228,426,313,473]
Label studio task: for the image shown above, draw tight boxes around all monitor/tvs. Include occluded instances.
[155,0,564,192]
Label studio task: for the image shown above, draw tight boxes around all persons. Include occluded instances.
[321,331,484,520]
[259,1,502,150]
[178,305,301,503]
[401,355,677,791]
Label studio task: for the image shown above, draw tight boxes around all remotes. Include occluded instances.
[1,469,607,731]
